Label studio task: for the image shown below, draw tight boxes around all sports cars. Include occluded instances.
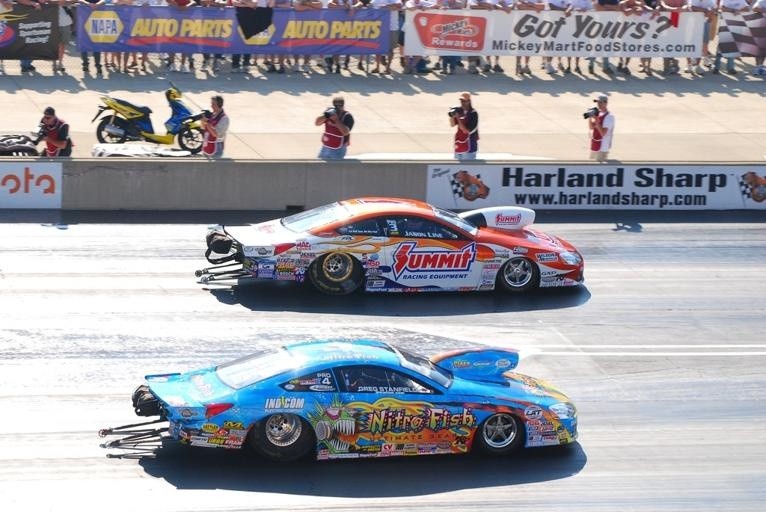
[98,338,579,463]
[193,197,584,296]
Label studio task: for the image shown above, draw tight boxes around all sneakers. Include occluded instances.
[703,59,712,67]
[695,67,705,75]
[712,67,719,74]
[493,64,503,73]
[622,66,630,74]
[266,66,275,73]
[467,65,478,74]
[524,65,531,74]
[82,64,88,71]
[540,62,544,69]
[58,63,65,72]
[168,63,176,72]
[96,69,102,75]
[544,64,557,74]
[447,70,455,75]
[760,65,766,77]
[251,61,257,67]
[558,62,565,72]
[588,64,595,74]
[356,63,363,70]
[563,66,570,74]
[728,69,736,75]
[430,62,441,70]
[111,61,116,69]
[371,65,379,73]
[104,63,110,68]
[189,66,198,73]
[752,66,760,77]
[514,64,523,76]
[292,63,299,72]
[19,64,35,74]
[212,62,219,71]
[683,64,691,73]
[646,68,652,77]
[127,62,137,69]
[230,66,240,74]
[638,65,645,74]
[383,66,390,73]
[140,64,145,72]
[303,66,311,73]
[200,61,207,72]
[602,66,614,74]
[615,63,624,70]
[262,60,269,65]
[178,65,186,73]
[573,66,581,76]
[284,59,291,65]
[277,65,284,74]
[49,63,57,74]
[241,66,249,73]
[326,66,333,74]
[439,67,446,74]
[335,63,341,74]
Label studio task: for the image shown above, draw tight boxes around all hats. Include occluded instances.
[41,106,56,116]
[458,91,471,101]
[592,93,608,103]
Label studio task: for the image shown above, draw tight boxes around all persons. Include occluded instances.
[450,93,480,153]
[200,96,229,156]
[351,378,364,392]
[589,95,615,161]
[37,107,74,157]
[1,0,766,77]
[315,97,355,158]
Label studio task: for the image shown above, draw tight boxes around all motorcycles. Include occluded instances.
[91,81,207,153]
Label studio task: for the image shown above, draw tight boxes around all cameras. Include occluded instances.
[201,109,213,118]
[38,123,48,136]
[447,106,463,117]
[324,106,339,118]
[583,105,599,120]
